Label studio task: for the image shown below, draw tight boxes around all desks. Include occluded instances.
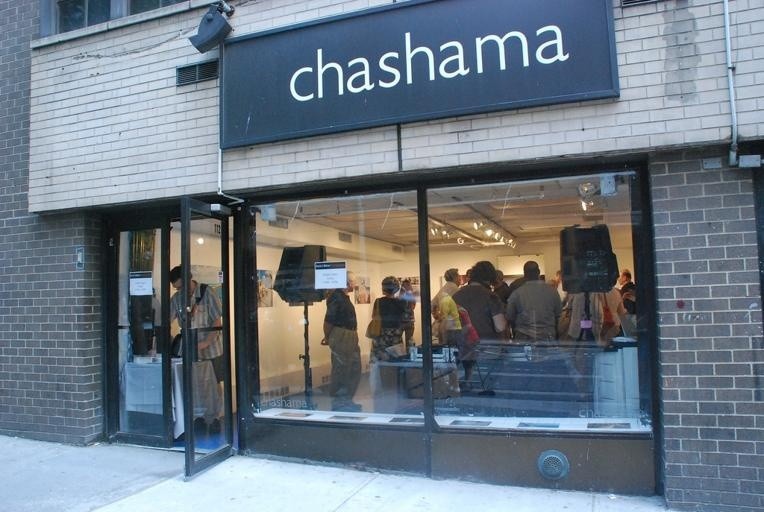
[126,359,214,444]
[372,360,460,414]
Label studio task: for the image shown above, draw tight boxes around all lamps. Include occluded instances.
[562,224,620,342]
[190,0,236,55]
[268,246,337,408]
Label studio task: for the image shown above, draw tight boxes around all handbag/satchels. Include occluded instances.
[602,306,614,329]
[364,298,383,339]
[169,331,183,357]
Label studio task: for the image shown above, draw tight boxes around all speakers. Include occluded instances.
[560,224,620,293]
[273,245,326,302]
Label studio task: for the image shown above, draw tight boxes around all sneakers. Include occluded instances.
[195,418,221,435]
[331,401,365,414]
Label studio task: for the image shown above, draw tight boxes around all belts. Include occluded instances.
[333,323,357,331]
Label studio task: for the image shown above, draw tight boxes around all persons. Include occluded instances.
[320,270,362,413]
[169,266,224,433]
[369,261,638,364]
